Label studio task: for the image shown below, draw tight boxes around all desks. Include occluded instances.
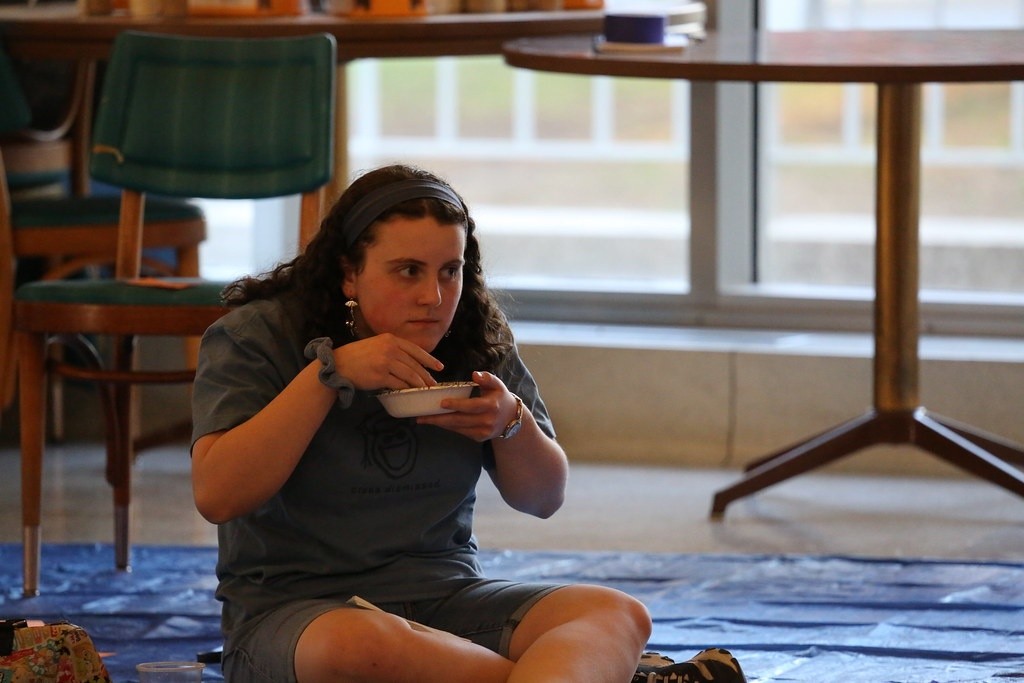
[500,25,1024,521]
[0,5,709,258]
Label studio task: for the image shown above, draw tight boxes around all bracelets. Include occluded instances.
[304,337,356,413]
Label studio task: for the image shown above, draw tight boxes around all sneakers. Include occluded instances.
[632,648,747,683]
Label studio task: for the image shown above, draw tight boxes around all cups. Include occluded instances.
[136,662,206,683]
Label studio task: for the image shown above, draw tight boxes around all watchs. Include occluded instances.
[495,392,524,439]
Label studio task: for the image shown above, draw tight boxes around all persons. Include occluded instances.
[189,165,748,682]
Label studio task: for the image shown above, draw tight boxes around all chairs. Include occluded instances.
[0,31,337,599]
[0,59,205,447]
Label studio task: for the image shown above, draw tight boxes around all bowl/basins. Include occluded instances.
[374,381,478,418]
